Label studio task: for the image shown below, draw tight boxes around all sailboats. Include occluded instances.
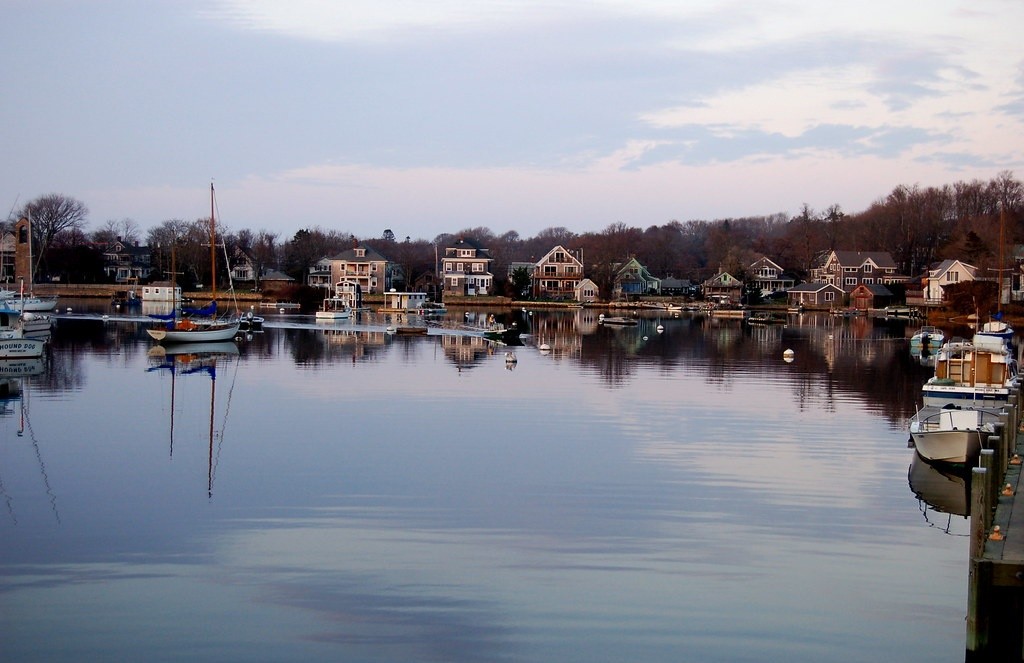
[146,182,242,345]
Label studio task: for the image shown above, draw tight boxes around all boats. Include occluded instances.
[315,298,350,318]
[482,324,522,346]
[909,340,1018,464]
[1,273,58,342]
[910,326,945,347]
[0,309,47,377]
[973,307,1014,344]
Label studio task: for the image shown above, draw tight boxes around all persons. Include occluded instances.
[488,313,497,329]
[920,334,931,359]
